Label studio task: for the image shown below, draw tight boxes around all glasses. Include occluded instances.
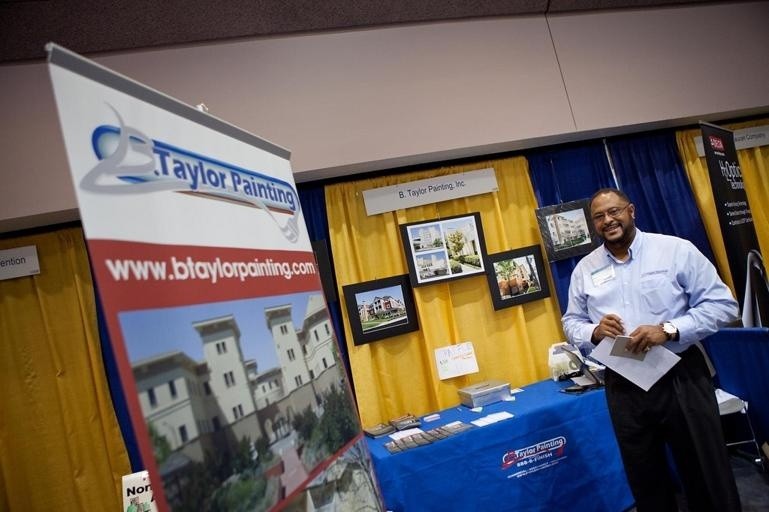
[592,204,630,224]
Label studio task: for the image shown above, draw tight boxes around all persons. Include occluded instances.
[558,185,742,512]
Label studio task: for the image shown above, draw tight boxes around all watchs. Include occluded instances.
[660,321,678,342]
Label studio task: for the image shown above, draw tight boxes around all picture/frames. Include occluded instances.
[483,245,550,311]
[399,212,487,288]
[535,197,602,264]
[342,275,419,346]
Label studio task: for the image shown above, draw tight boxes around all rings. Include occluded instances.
[646,345,651,351]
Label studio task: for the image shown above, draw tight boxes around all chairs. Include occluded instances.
[715,388,766,474]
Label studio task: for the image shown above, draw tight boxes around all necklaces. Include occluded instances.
[616,244,628,260]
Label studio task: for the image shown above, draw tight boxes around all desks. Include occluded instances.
[364,379,637,512]
